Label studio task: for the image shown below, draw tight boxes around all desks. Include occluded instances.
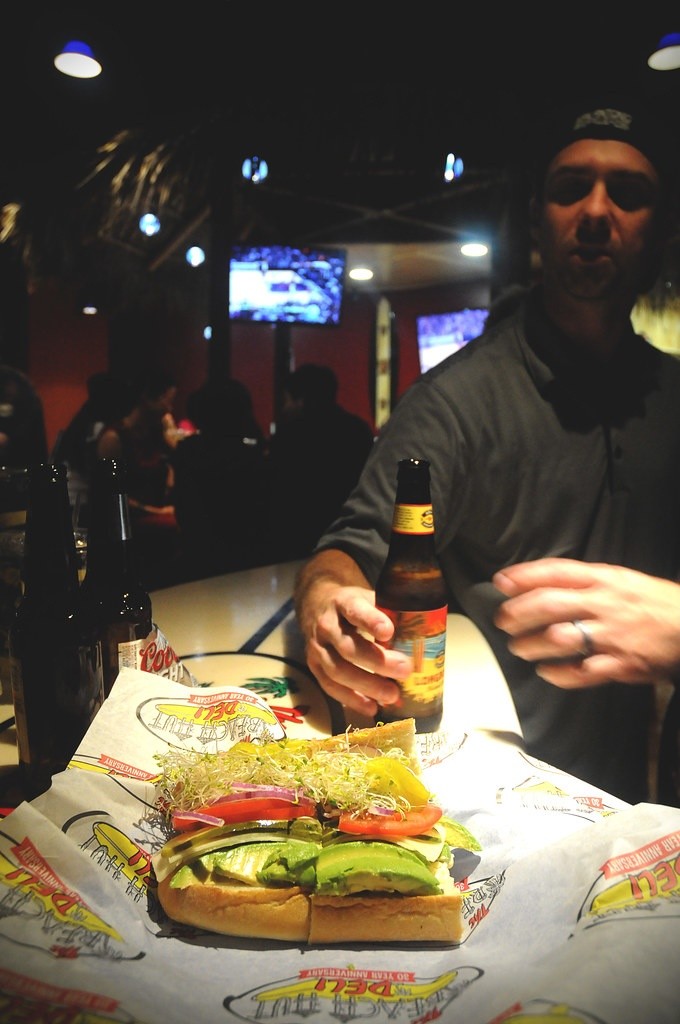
[0,544,528,1024]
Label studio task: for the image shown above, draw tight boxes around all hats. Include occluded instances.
[533,99,670,211]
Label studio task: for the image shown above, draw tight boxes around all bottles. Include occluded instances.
[374,458,448,736]
[7,466,105,801]
[82,458,152,699]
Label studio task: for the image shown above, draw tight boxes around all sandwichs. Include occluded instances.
[147,715,481,943]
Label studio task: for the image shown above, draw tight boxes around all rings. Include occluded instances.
[571,618,594,658]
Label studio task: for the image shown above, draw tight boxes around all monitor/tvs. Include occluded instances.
[416,307,490,374]
[223,241,346,329]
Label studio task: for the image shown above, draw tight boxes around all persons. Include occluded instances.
[492,556,680,809]
[0,340,376,594]
[291,105,680,806]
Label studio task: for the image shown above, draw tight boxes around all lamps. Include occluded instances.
[646,30,680,72]
[53,42,102,78]
